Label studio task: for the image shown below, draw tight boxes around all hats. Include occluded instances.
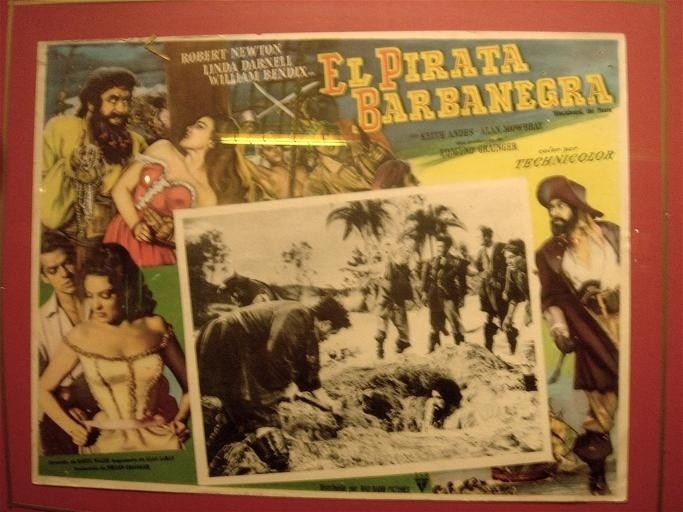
[536,174,604,218]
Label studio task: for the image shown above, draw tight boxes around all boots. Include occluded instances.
[572,430,612,496]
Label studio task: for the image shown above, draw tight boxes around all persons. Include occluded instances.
[37,68,143,269]
[104,111,223,266]
[532,174,625,497]
[36,244,191,455]
[34,230,98,454]
[224,94,419,201]
[374,223,530,360]
[193,298,352,469]
